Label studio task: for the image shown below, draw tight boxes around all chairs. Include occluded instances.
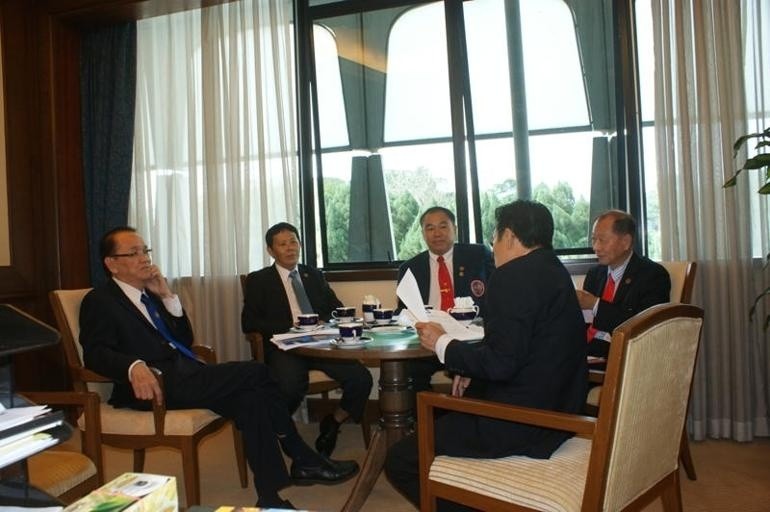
[588,260,699,481]
[416,301,705,512]
[50,286,248,507]
[241,274,372,451]
[4,387,103,507]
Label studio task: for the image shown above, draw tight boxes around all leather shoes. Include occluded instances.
[254,500,296,509]
[290,454,360,488]
[315,414,341,457]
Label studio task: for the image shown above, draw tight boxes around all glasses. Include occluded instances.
[113,248,153,258]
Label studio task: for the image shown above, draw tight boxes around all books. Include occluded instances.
[394,267,484,344]
[0,401,64,471]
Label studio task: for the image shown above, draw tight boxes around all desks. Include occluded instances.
[275,314,483,512]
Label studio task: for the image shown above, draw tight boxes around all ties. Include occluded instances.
[585,274,616,343]
[436,256,454,311]
[289,271,313,315]
[141,294,193,360]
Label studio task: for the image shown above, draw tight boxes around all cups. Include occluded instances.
[340,322,363,342]
[373,308,392,324]
[332,307,356,323]
[294,313,319,329]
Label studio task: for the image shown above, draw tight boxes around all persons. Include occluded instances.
[382,198,591,511]
[575,209,673,388]
[78,226,361,510]
[396,206,497,420]
[241,221,374,459]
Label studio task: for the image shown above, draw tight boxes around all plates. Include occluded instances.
[329,317,365,322]
[290,325,324,332]
[329,337,373,349]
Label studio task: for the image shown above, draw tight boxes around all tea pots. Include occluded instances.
[362,294,382,322]
